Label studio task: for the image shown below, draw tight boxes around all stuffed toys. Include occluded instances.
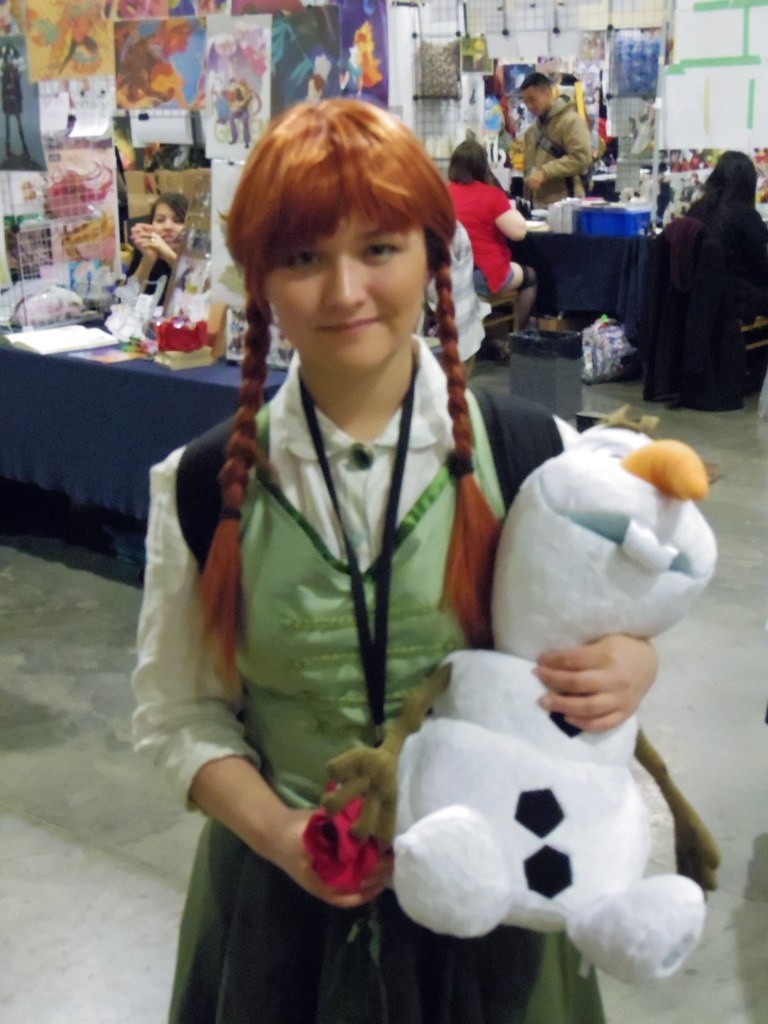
[317,413,729,991]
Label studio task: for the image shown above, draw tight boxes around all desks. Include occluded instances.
[2,319,281,526]
[508,224,660,313]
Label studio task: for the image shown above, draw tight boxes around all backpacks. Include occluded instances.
[533,119,595,193]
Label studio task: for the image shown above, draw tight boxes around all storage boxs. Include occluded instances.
[572,207,652,237]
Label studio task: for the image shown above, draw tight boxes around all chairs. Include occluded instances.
[425,219,518,372]
[643,217,768,412]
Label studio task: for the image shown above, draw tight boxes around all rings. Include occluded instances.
[149,238,156,243]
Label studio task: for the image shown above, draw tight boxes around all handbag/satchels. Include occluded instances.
[582,319,637,380]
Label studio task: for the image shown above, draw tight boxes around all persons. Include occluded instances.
[669,147,766,414]
[515,73,597,207]
[119,192,214,307]
[118,90,666,1024]
[429,136,546,351]
